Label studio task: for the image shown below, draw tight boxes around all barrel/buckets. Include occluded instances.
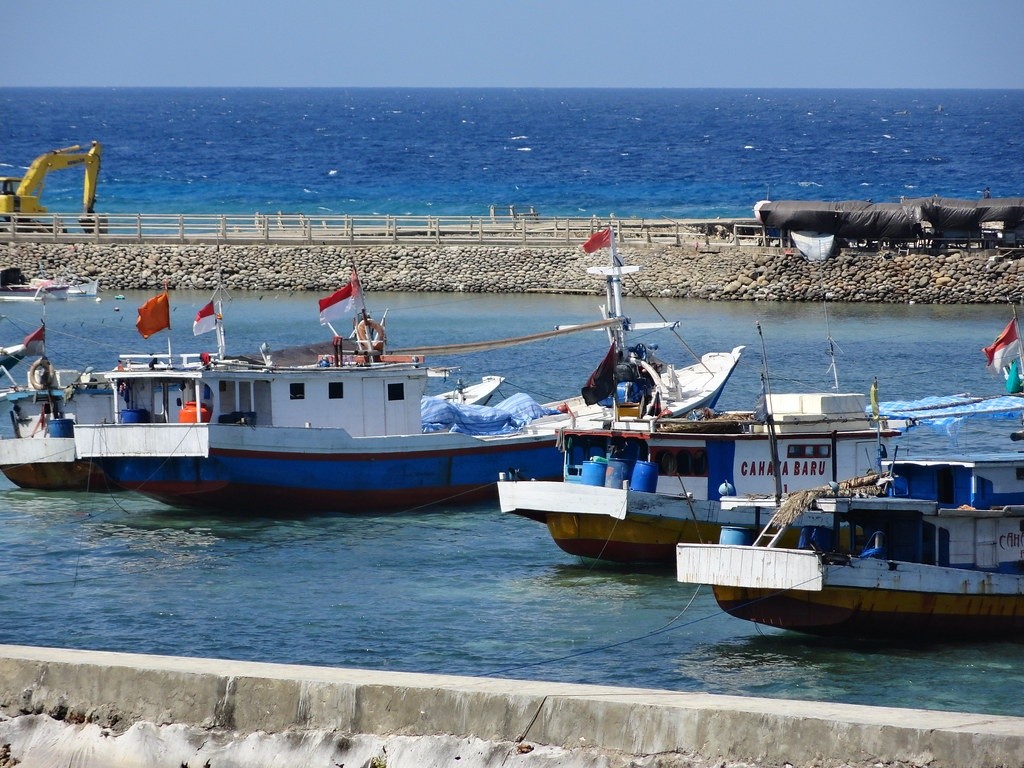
[629,459,658,494]
[178,401,211,423]
[120,409,148,424]
[718,526,751,547]
[580,460,607,485]
[48,419,74,438]
[605,456,632,489]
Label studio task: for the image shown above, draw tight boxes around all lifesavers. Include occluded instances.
[357,319,384,351]
[30,359,55,390]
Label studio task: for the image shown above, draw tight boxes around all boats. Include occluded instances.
[676,376,1024,641]
[497,214,914,563]
[0,259,750,522]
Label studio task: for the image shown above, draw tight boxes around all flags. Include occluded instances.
[24,325,45,356]
[981,319,1020,374]
[318,270,365,326]
[870,384,879,422]
[136,292,170,339]
[581,341,615,407]
[193,301,217,336]
[583,228,611,253]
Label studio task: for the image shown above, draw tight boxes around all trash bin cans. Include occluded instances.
[218,411,256,426]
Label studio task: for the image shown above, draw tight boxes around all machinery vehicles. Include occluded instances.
[0,138,112,235]
